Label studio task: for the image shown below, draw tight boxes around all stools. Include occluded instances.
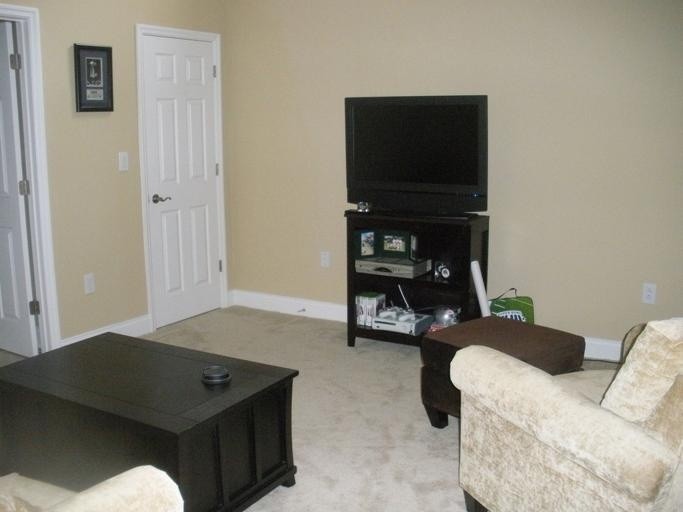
[421,314,589,439]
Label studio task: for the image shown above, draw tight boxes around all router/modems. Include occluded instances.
[372,283,433,337]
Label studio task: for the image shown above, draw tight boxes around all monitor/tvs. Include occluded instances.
[344,96,487,219]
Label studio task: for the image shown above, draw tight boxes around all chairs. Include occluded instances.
[0,462,188,512]
[448,318,683,512]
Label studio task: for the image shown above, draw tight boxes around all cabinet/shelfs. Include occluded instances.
[340,207,499,348]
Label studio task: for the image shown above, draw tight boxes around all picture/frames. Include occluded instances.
[71,41,117,114]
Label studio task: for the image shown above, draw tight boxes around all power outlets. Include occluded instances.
[641,281,657,306]
[84,272,95,295]
